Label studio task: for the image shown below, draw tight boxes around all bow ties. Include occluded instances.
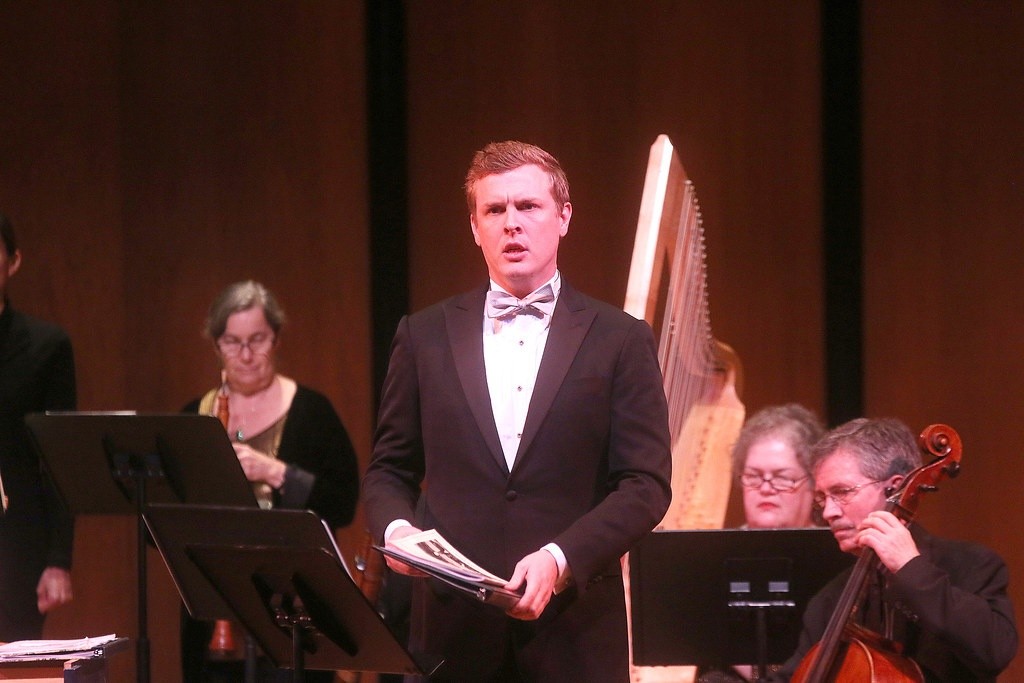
[487,282,557,317]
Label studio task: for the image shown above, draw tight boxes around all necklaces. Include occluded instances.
[233,427,246,441]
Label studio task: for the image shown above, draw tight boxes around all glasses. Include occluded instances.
[738,472,809,491]
[216,334,277,355]
[813,478,889,507]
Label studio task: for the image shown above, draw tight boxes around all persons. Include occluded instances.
[691,404,1017,683]
[0,218,78,644]
[361,140,673,683]
[177,280,360,683]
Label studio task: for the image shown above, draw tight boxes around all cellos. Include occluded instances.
[788,422,963,683]
